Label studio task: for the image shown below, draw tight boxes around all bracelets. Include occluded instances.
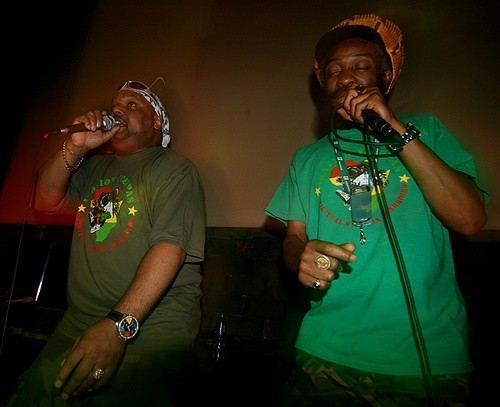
[63,138,84,171]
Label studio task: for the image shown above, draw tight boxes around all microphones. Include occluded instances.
[361,108,404,146]
[41,115,116,140]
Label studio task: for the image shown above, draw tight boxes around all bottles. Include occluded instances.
[214,313,226,351]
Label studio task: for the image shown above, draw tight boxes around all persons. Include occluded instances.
[263,14,490,407]
[4,80,208,407]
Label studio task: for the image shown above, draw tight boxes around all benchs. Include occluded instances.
[0,223,500,407]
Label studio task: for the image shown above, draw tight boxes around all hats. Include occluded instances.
[117,77,171,148]
[313,14,405,95]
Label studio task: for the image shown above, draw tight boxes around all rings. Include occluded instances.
[92,368,104,381]
[314,255,331,271]
[312,279,321,290]
[355,84,365,96]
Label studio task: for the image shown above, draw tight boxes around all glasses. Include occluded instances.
[117,80,164,111]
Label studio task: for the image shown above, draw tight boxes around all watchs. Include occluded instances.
[393,122,422,151]
[105,310,140,343]
